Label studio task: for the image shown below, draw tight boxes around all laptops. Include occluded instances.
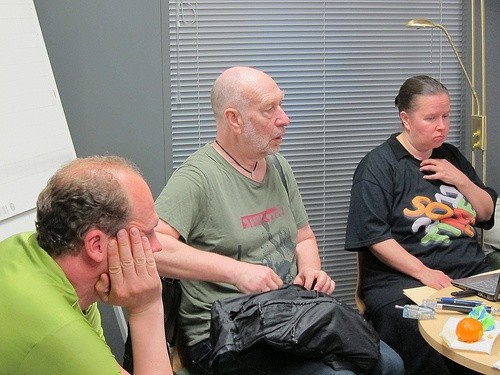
[450,272,500,302]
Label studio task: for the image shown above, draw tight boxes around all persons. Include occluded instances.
[345,76,500,375]
[153,66,404,375]
[0,155,175,375]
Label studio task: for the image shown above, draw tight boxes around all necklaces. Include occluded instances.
[215,140,258,179]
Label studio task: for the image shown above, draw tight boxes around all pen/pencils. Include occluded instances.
[436,296,486,306]
[437,303,500,314]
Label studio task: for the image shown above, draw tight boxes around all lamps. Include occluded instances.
[407,1,486,185]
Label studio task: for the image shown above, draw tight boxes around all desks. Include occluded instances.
[417,270,500,375]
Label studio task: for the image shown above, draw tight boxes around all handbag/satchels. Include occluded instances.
[208,284,380,375]
